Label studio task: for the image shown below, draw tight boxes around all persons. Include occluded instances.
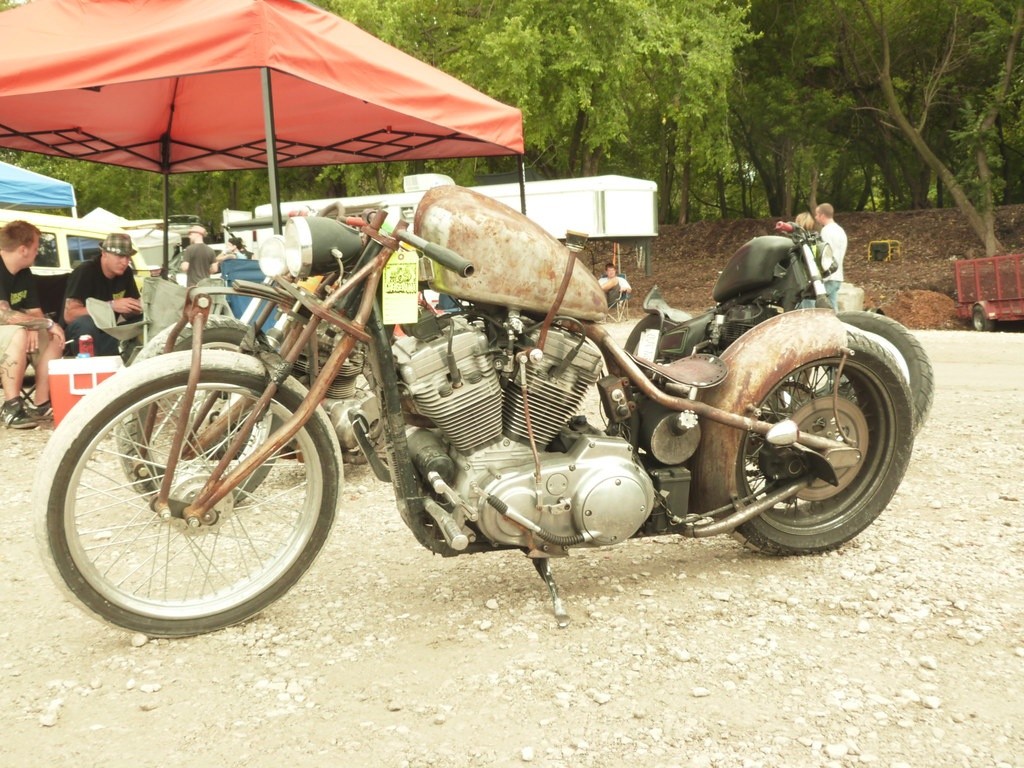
[596,264,631,308]
[215,237,248,263]
[57,232,144,357]
[0,221,67,428]
[814,203,848,312]
[795,212,816,310]
[179,225,218,288]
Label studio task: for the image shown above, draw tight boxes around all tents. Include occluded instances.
[0,0,527,279]
[0,160,85,263]
[81,205,181,269]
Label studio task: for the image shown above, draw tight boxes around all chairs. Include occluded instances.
[599,273,629,324]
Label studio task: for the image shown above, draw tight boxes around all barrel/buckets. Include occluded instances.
[48,356,125,430]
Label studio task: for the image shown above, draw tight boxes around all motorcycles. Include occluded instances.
[625,212,935,435]
[32,203,917,638]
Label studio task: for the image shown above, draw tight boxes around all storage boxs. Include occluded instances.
[48,355,125,429]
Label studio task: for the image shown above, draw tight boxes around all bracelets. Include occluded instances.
[45,318,54,331]
[110,299,113,307]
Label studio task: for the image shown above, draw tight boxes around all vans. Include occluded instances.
[1,210,151,292]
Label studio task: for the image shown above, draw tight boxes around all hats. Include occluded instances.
[187,225,208,237]
[98,233,138,257]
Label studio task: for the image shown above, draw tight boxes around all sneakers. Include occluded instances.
[0,396,38,429]
[27,400,54,420]
